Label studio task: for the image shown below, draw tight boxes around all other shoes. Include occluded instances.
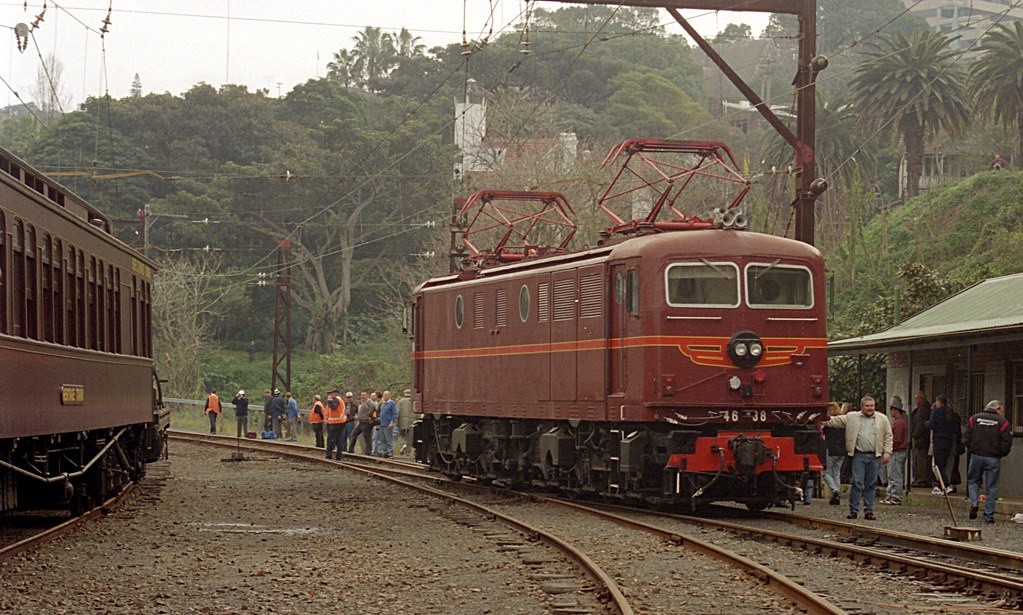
[828,491,840,505]
[399,444,407,456]
[864,512,876,520]
[847,511,858,519]
[945,486,958,494]
[931,486,946,496]
[775,503,789,508]
[969,504,979,520]
[985,518,994,523]
[878,495,901,505]
[803,502,811,505]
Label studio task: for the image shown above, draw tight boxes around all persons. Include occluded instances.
[879,392,965,504]
[263,388,298,441]
[308,388,421,460]
[775,402,852,507]
[232,390,248,437]
[961,400,1012,521]
[992,153,1006,170]
[812,396,893,519]
[246,341,255,361]
[204,388,221,433]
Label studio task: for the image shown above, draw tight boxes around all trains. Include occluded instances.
[1,144,169,519]
[403,138,835,512]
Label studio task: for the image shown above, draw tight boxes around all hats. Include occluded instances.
[238,390,245,395]
[324,387,340,393]
[346,392,353,399]
[274,389,281,395]
[403,388,412,397]
[890,396,902,410]
